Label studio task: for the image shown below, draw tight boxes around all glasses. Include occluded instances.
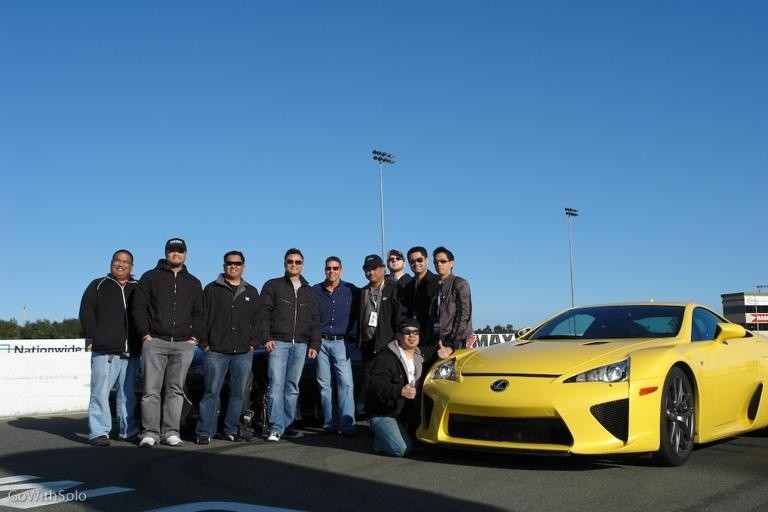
[285,259,303,265]
[224,261,244,266]
[397,328,420,336]
[434,260,451,265]
[326,267,341,270]
[409,258,426,264]
[387,257,404,261]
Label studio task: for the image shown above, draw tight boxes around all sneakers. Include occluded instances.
[195,435,211,445]
[161,436,184,446]
[221,434,239,441]
[88,435,110,446]
[284,431,305,438]
[266,432,281,442]
[121,434,141,443]
[139,436,156,447]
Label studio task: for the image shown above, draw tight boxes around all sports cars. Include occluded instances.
[416,303,768,465]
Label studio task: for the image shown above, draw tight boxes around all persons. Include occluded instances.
[194,251,260,445]
[404,246,442,345]
[386,249,412,318]
[134,238,203,449]
[364,318,455,457]
[259,248,322,442]
[311,256,359,436]
[79,249,139,447]
[428,246,473,350]
[359,254,403,359]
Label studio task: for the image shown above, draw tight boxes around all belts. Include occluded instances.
[321,335,344,340]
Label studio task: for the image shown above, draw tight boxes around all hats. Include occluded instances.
[363,254,385,269]
[387,249,405,262]
[392,318,420,333]
[165,237,187,251]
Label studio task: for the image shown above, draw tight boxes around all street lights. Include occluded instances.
[565,207,578,336]
[564,207,579,309]
